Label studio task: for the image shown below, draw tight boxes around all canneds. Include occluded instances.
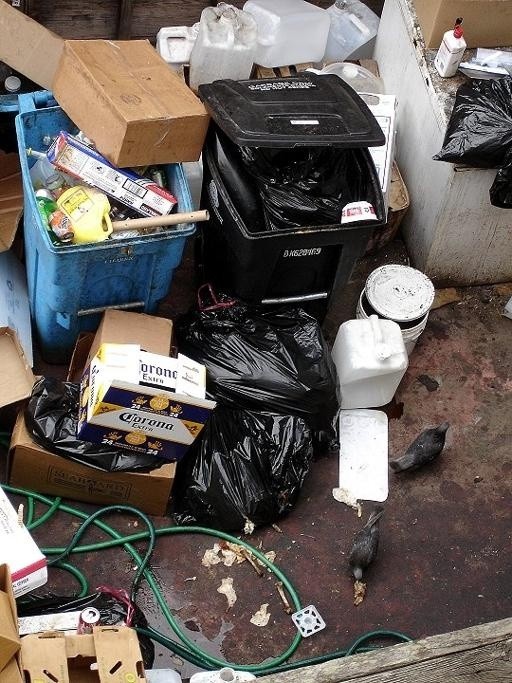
[46,173,72,200]
[49,209,74,244]
[151,169,168,188]
[77,606,103,634]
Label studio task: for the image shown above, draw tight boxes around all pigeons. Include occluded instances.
[389,422,450,474]
[348,504,386,584]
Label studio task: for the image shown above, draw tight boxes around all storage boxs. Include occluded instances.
[0,0,512,683]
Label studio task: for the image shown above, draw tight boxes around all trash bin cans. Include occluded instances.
[0,89,197,365]
[194,74,386,328]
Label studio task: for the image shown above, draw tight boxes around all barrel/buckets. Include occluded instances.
[243,0,330,68]
[190,4,258,91]
[355,264,436,359]
[56,185,114,243]
[330,314,408,410]
[315,1,381,70]
[157,19,200,69]
